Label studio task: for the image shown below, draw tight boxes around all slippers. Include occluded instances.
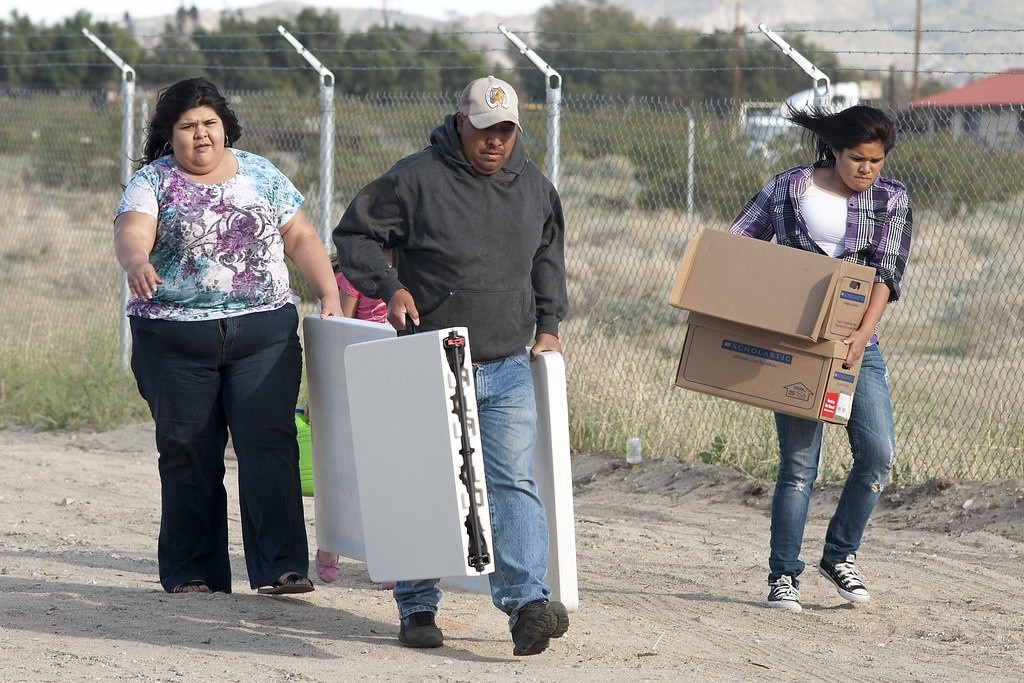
[258,573,315,594]
[170,581,212,594]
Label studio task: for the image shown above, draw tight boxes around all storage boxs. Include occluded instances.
[666,227,877,427]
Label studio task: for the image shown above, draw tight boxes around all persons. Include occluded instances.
[332,76,570,657]
[305,239,399,589]
[727,105,913,611]
[112,77,344,595]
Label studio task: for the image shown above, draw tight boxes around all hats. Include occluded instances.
[459,75,524,134]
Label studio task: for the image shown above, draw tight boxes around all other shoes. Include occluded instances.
[315,549,340,583]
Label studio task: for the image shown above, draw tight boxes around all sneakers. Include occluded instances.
[398,612,444,650]
[512,600,569,657]
[819,557,870,603]
[766,575,803,612]
